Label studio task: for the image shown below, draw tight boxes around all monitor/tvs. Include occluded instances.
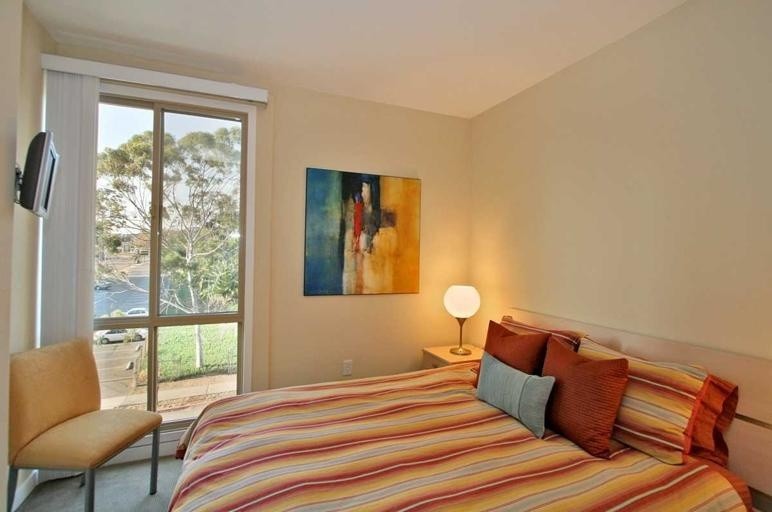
[19,130,61,220]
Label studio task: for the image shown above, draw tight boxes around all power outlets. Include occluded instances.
[343,359,353,376]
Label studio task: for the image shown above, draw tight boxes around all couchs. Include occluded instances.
[7,336,163,512]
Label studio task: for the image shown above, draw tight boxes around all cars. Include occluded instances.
[93,328,146,344]
[95,281,110,290]
[127,308,148,316]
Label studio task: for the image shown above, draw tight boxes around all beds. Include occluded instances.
[173,306,772,511]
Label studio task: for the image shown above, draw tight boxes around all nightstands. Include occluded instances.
[421,343,486,370]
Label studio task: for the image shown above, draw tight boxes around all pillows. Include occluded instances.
[475,320,710,466]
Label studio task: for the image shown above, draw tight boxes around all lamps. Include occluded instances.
[444,285,481,355]
